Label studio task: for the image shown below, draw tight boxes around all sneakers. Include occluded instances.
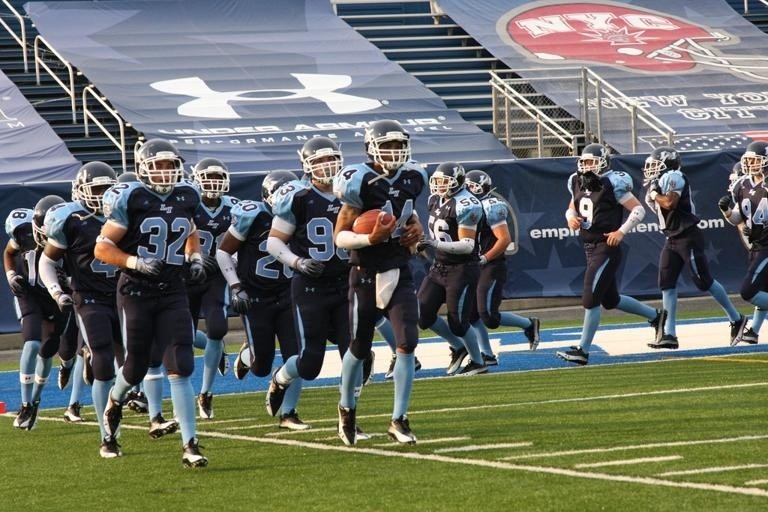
[387,414,418,445]
[181,437,209,467]
[480,351,498,365]
[81,344,95,386]
[121,390,141,408]
[647,334,680,350]
[384,353,422,380]
[103,384,124,437]
[362,350,376,387]
[337,401,359,447]
[526,315,541,351]
[57,355,78,390]
[455,361,488,377]
[445,345,469,375]
[127,391,149,413]
[741,328,759,344]
[555,344,590,366]
[649,308,668,344]
[147,413,180,440]
[728,312,748,348]
[233,342,255,381]
[356,424,373,441]
[64,402,85,423]
[21,398,41,431]
[196,391,215,419]
[99,437,123,459]
[279,407,311,431]
[265,366,291,417]
[214,338,231,376]
[12,402,30,427]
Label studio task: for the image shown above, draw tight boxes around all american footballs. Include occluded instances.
[352,209,397,237]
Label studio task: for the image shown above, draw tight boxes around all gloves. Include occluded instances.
[189,258,207,283]
[717,193,733,217]
[649,179,661,201]
[293,258,325,280]
[56,294,74,312]
[416,238,438,253]
[8,275,27,297]
[135,255,165,277]
[231,287,252,315]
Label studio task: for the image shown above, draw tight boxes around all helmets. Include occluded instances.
[641,145,683,181]
[428,159,467,198]
[133,137,186,196]
[296,135,345,186]
[31,194,68,249]
[260,167,300,205]
[71,160,120,210]
[576,143,611,176]
[740,140,768,177]
[363,119,413,176]
[190,156,231,200]
[464,169,493,200]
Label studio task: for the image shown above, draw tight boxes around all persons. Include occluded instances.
[4,196,84,428]
[38,161,178,457]
[556,144,667,365]
[265,135,367,442]
[215,173,312,431]
[332,120,430,446]
[718,139,764,347]
[413,163,484,375]
[94,139,209,469]
[465,171,540,365]
[180,159,241,418]
[644,147,749,349]
